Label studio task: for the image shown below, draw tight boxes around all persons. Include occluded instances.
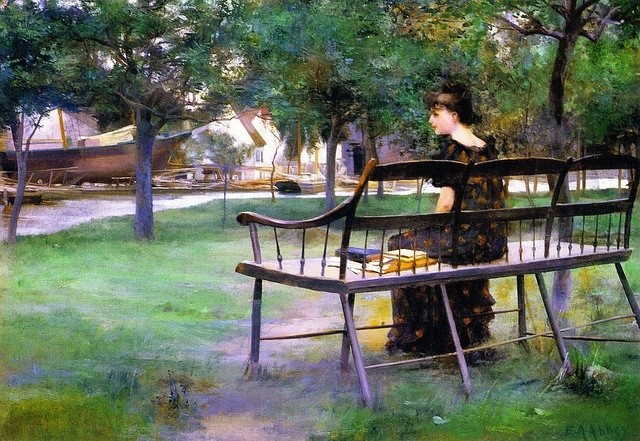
[384,80,510,359]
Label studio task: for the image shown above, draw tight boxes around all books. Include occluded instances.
[383,249,427,264]
[385,249,426,258]
[334,246,382,264]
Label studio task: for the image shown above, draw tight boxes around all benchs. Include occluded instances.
[235,153,639,411]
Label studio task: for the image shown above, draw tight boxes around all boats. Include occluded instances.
[0,132,192,184]
